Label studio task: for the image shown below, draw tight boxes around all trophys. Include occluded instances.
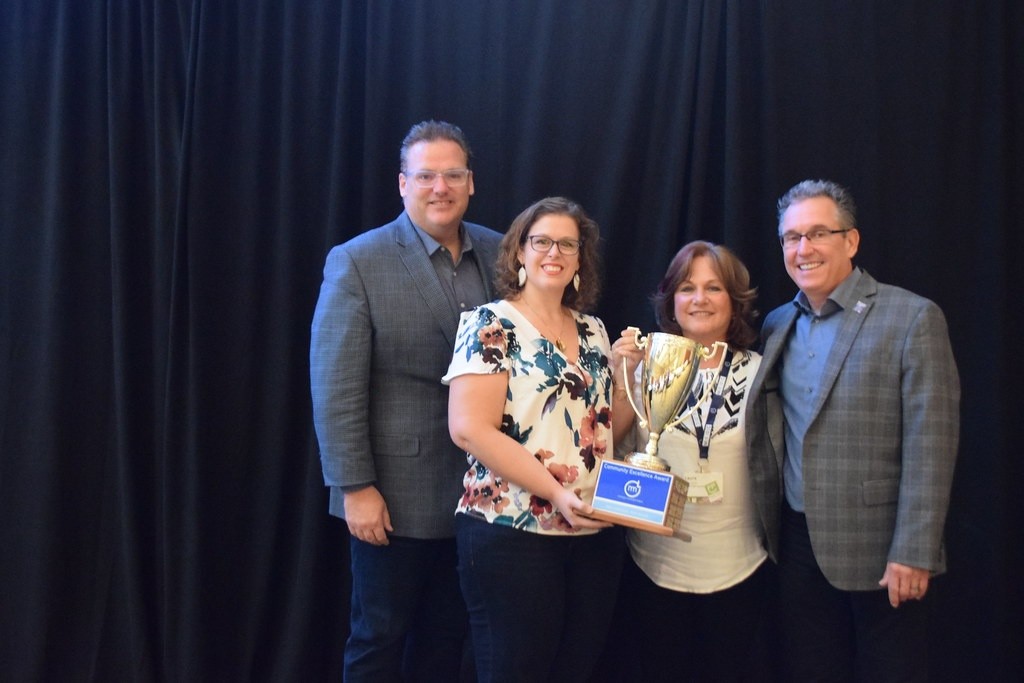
[575,326,727,542]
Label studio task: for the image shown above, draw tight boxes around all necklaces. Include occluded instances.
[521,294,568,352]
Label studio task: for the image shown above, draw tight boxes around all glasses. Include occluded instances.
[779,229,849,248]
[403,169,470,188]
[525,234,584,256]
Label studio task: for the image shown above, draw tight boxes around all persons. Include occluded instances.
[308,121,519,683]
[440,197,616,683]
[746,179,960,683]
[606,240,774,683]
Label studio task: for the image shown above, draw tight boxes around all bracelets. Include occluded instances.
[612,378,633,401]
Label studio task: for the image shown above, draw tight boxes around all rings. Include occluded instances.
[911,585,919,590]
